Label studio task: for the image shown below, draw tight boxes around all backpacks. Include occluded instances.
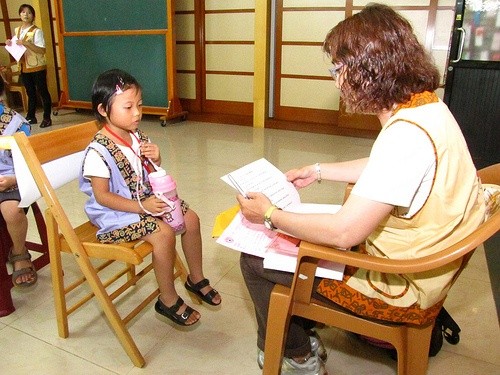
[388,305,461,362]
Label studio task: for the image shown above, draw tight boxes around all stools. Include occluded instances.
[0,200,66,318]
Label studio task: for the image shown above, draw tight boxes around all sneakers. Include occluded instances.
[258,348,329,375]
[308,330,327,361]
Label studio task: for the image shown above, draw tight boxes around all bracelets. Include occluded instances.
[315,162,321,183]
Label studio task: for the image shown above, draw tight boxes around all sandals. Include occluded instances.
[8,246,38,291]
[184,275,222,306]
[154,294,200,326]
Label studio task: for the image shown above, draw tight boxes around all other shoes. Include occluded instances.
[25,116,37,124]
[40,119,51,128]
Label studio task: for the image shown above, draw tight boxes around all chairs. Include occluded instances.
[14,118,202,369]
[0,60,29,115]
[263,163,500,375]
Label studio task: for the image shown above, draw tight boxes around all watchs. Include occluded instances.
[264,205,283,231]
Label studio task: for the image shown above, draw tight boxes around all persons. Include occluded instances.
[0,76,37,288]
[237,3,486,375]
[5,4,52,129]
[79,68,222,326]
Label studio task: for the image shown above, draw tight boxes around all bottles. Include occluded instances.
[148,169,186,236]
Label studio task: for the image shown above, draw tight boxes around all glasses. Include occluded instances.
[327,62,347,79]
